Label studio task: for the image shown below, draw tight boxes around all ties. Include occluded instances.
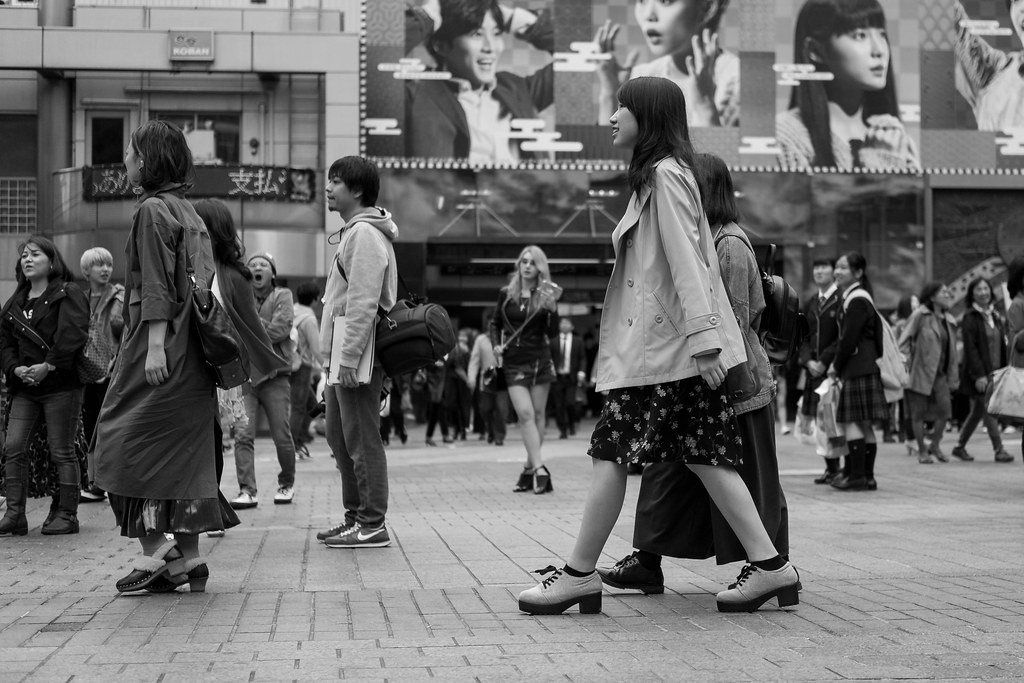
[561,337,566,368]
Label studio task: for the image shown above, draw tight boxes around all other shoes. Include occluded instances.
[883,430,1015,464]
[814,468,876,491]
[382,424,506,448]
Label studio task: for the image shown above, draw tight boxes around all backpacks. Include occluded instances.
[288,313,310,373]
[716,232,806,366]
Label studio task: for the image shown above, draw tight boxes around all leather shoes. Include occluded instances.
[595,551,664,594]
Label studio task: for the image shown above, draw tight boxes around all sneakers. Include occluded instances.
[230,492,258,509]
[325,522,391,548]
[317,522,351,540]
[274,486,294,504]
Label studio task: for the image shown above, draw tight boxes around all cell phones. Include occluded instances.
[540,281,563,301]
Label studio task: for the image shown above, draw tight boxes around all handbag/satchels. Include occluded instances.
[982,330,1024,425]
[375,297,454,376]
[190,287,251,390]
[482,367,507,390]
[63,281,113,381]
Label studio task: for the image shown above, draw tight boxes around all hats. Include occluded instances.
[246,252,276,274]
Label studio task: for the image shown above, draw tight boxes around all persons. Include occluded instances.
[380,375,408,446]
[191,198,289,536]
[315,155,399,548]
[80,247,125,501]
[550,318,600,438]
[592,0,740,128]
[594,146,790,593]
[515,75,800,614]
[802,251,892,491]
[404,0,554,159]
[0,232,91,535]
[889,281,961,464]
[776,0,921,222]
[88,119,243,592]
[951,254,1024,464]
[944,0,1024,129]
[229,250,294,507]
[489,244,561,494]
[409,310,496,446]
[292,276,324,462]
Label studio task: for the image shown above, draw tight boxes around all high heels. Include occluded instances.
[145,558,209,591]
[518,565,602,615]
[514,466,536,492]
[717,561,799,611]
[534,465,553,494]
[116,538,185,592]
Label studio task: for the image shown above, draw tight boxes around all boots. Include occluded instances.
[41,481,80,535]
[0,479,28,535]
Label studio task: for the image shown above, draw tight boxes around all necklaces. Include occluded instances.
[519,293,530,312]
